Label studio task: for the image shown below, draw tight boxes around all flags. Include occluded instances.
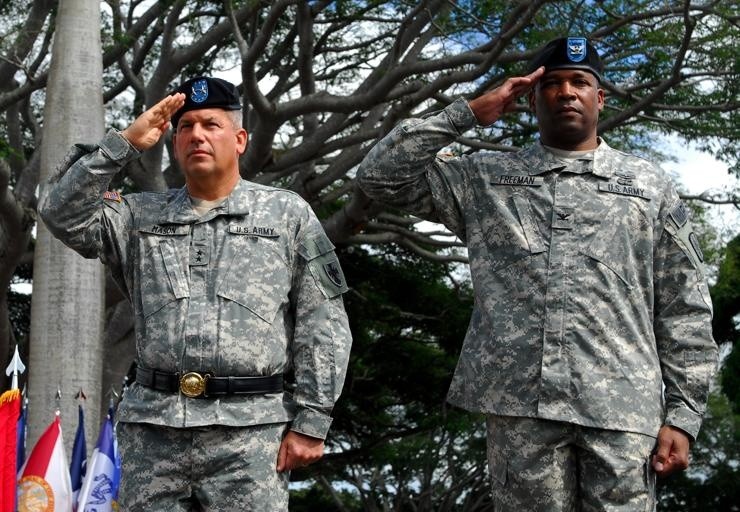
[15,386,73,512]
[16,382,30,512]
[75,382,121,512]
[69,387,89,512]
[0,343,26,512]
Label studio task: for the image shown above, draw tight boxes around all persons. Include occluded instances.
[353,31,721,512]
[36,76,356,512]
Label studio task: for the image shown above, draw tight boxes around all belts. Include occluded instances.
[135,364,287,400]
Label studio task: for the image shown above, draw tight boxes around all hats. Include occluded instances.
[530,36,605,84]
[167,77,243,128]
